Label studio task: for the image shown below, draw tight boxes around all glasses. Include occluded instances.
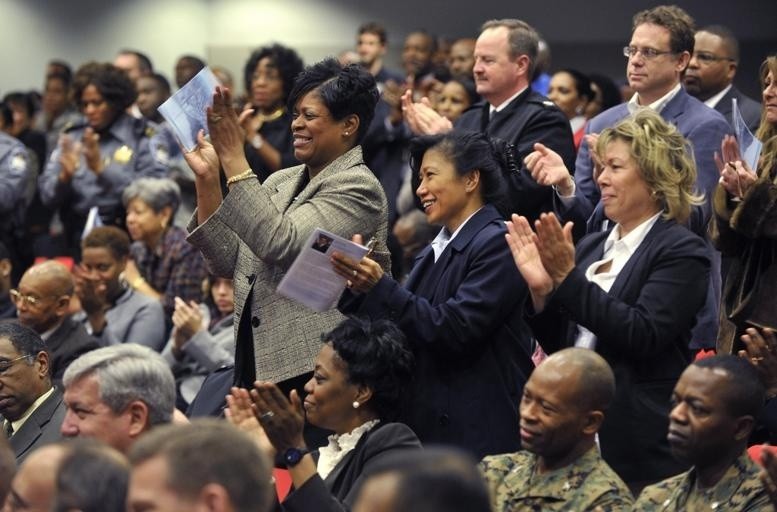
[8,289,59,310]
[622,46,671,59]
[692,50,737,69]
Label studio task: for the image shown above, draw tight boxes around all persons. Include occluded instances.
[1,4,777,510]
